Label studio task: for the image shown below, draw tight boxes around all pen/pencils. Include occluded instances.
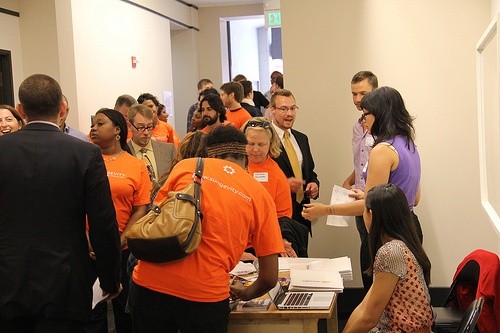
[228,275,237,286]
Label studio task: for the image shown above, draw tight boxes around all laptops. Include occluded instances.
[253,258,336,309]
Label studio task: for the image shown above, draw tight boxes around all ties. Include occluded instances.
[139,148,156,182]
[283,131,304,204]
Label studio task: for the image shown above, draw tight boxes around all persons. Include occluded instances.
[302,86,423,256]
[342,71,380,294]
[341,185,434,333]
[0,71,320,333]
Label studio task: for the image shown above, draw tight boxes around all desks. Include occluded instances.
[226,270,339,332]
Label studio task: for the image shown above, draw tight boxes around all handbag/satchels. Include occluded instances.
[125,156,203,263]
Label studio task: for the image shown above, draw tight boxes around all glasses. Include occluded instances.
[276,106,299,113]
[362,112,372,121]
[244,121,274,139]
[129,120,155,132]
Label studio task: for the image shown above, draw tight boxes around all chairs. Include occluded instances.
[429,249,499,331]
[435,298,484,333]
